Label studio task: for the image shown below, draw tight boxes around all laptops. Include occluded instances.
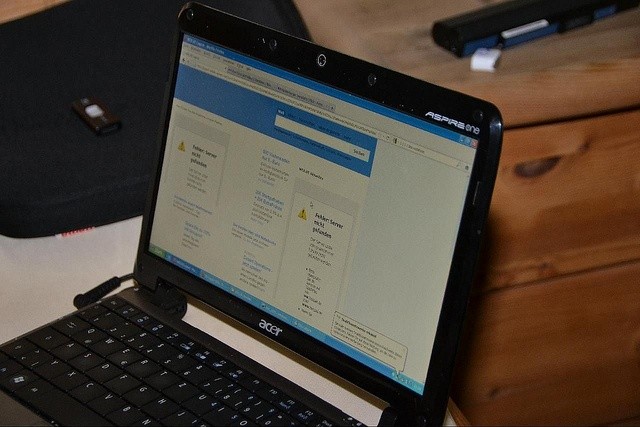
[0,0,503,427]
